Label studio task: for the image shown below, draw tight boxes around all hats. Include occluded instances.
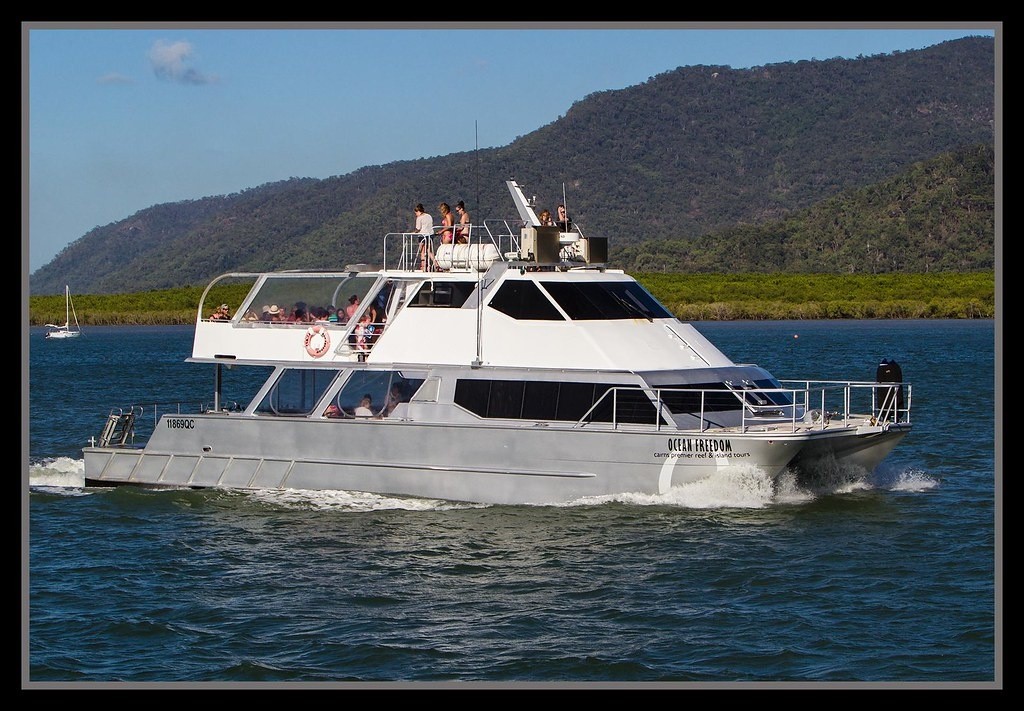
[268,304,280,313]
[262,305,270,313]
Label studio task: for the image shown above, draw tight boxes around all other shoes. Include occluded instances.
[435,266,443,271]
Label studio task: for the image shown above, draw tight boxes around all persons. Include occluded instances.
[323,379,412,418]
[208,302,350,326]
[347,279,395,353]
[412,203,443,272]
[555,204,572,232]
[538,209,554,226]
[438,203,454,244]
[456,201,471,244]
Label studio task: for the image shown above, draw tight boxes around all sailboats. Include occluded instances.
[44,285,80,338]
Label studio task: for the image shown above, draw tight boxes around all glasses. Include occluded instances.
[456,207,460,212]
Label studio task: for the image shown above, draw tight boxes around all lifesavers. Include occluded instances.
[305,325,329,357]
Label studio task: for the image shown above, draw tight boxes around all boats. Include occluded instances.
[81,181,912,507]
